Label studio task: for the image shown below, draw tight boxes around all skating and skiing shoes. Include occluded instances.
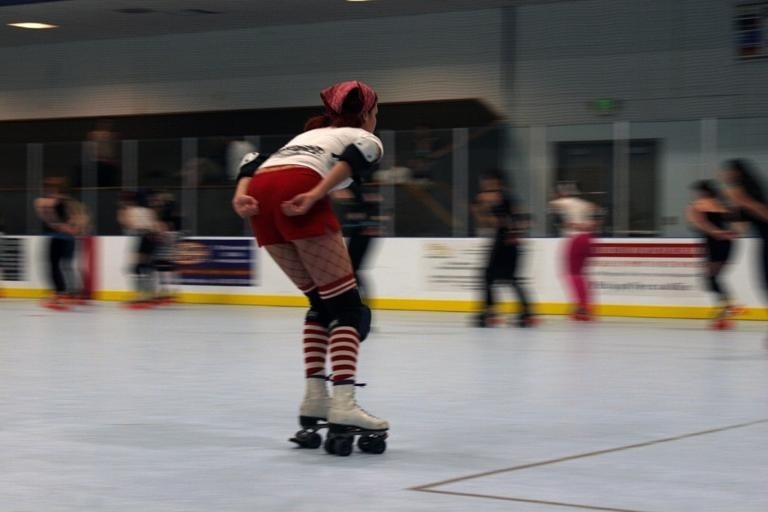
[287,374,330,450]
[324,379,389,457]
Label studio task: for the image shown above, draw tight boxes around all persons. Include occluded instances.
[550,177,602,320]
[233,79,392,458]
[695,156,768,290]
[466,166,538,326]
[684,178,743,334]
[115,188,167,311]
[330,187,372,302]
[32,174,96,310]
